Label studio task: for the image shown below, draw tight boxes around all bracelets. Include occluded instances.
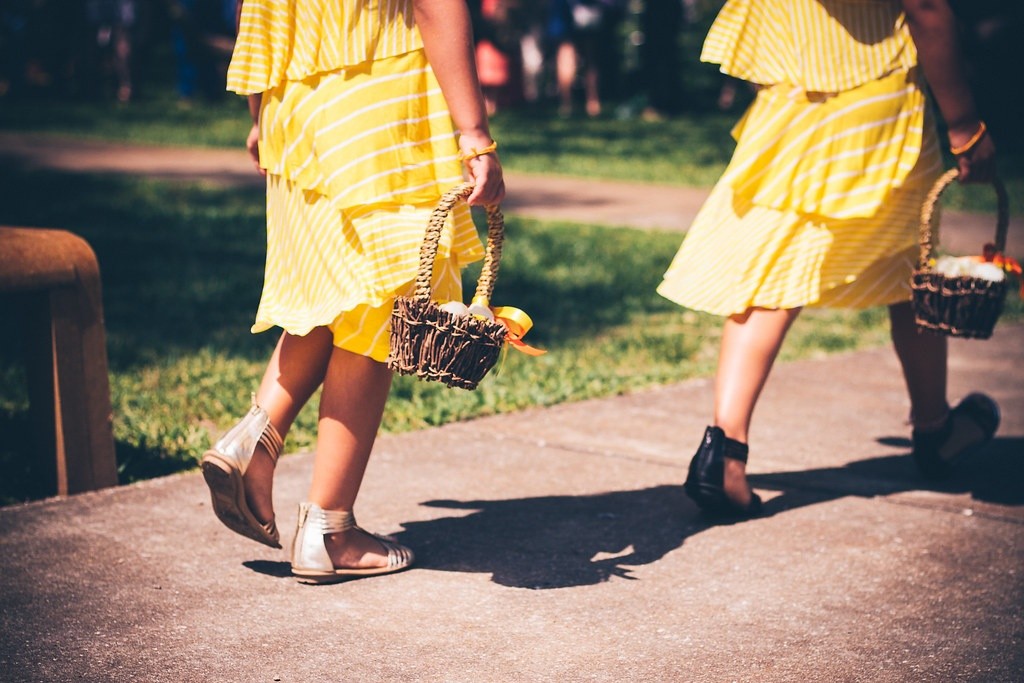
[456,139,497,160]
[951,120,985,155]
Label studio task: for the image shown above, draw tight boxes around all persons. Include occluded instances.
[658,0,1002,513]
[201,0,506,584]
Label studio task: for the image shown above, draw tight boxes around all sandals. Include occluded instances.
[200,391,282,550]
[913,392,1000,483]
[290,503,415,584]
[683,426,763,512]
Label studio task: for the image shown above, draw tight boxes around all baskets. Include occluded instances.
[910,166,1022,340]
[386,182,510,391]
